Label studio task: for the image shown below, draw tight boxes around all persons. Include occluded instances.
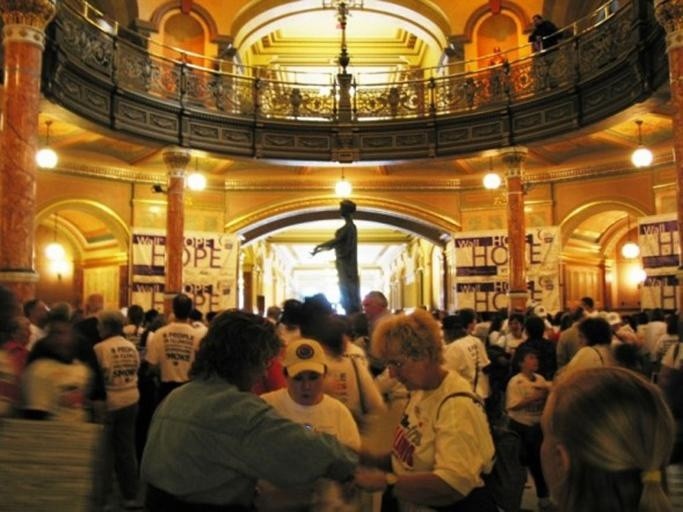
[309,199,362,319]
[0,282,682,512]
[527,14,563,91]
[489,47,513,93]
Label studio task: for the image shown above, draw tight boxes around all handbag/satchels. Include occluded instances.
[492,425,528,512]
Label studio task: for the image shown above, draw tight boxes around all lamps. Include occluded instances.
[45,210,65,262]
[629,117,652,170]
[481,155,501,194]
[34,120,58,170]
[335,168,352,198]
[185,157,208,192]
[620,212,639,261]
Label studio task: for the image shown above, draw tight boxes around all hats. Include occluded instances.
[285,339,327,378]
